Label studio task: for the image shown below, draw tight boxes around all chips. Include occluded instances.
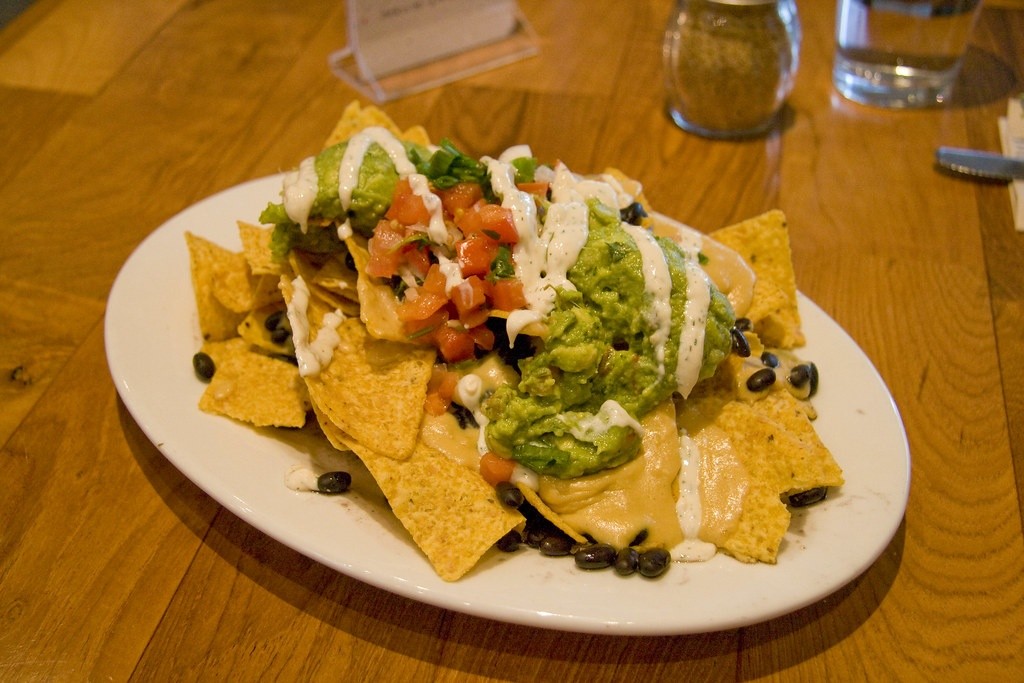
[182,99,845,582]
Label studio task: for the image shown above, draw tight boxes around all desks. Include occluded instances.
[0,1,1024,683]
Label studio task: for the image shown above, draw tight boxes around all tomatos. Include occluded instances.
[366,178,554,485]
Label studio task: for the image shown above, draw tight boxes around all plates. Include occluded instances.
[105,171,912,634]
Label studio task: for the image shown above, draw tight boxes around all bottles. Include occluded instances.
[660,0,802,140]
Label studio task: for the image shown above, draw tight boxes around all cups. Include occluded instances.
[832,0,983,109]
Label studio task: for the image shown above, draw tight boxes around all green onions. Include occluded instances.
[396,137,537,205]
